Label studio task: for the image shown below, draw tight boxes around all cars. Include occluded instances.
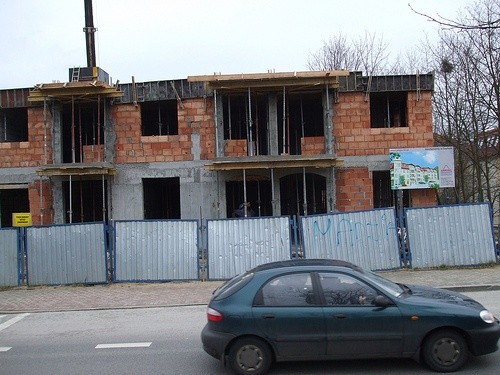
[199,258,500,375]
